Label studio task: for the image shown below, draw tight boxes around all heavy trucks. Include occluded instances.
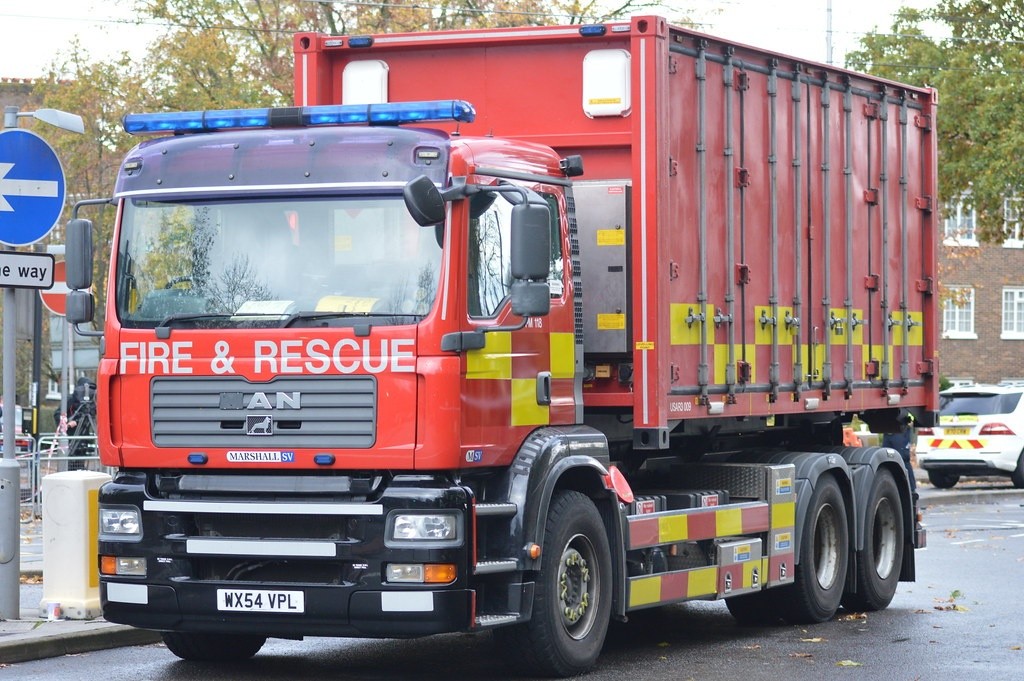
[64,16,938,679]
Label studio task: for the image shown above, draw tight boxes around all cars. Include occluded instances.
[1,403,30,451]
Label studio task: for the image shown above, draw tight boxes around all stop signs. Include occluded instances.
[40,263,88,317]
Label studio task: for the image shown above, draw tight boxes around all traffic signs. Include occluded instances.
[1,251,54,288]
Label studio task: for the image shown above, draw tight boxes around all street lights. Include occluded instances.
[1,106,84,620]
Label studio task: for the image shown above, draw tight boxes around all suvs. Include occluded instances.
[916,382,1024,491]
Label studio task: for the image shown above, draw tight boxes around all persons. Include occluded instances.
[54,389,97,470]
